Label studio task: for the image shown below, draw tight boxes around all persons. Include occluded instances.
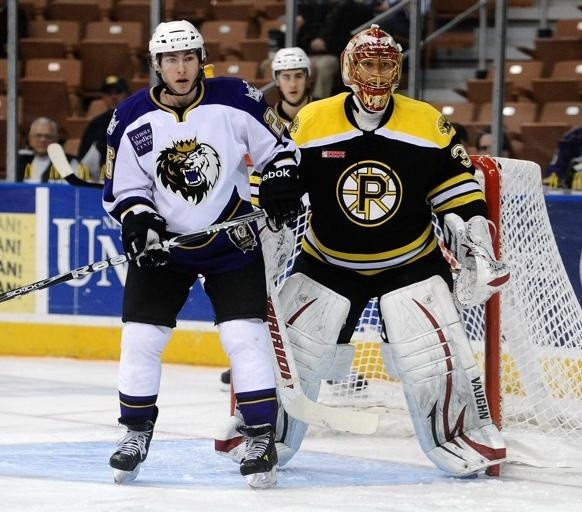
[450,120,468,159]
[223,45,367,393]
[219,22,509,478]
[259,1,430,102]
[100,18,305,475]
[538,121,582,187]
[80,76,132,180]
[473,125,515,161]
[14,117,78,181]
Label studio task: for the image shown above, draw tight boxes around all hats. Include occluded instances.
[97,75,130,93]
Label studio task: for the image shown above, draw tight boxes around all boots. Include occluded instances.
[221,363,232,385]
[234,421,279,476]
[107,406,162,472]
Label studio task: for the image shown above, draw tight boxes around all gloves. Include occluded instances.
[259,161,304,232]
[121,213,171,270]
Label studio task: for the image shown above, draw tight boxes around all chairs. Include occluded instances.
[0,0,582,194]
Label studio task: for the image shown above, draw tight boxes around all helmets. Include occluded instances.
[340,23,405,112]
[270,46,314,81]
[148,20,209,73]
